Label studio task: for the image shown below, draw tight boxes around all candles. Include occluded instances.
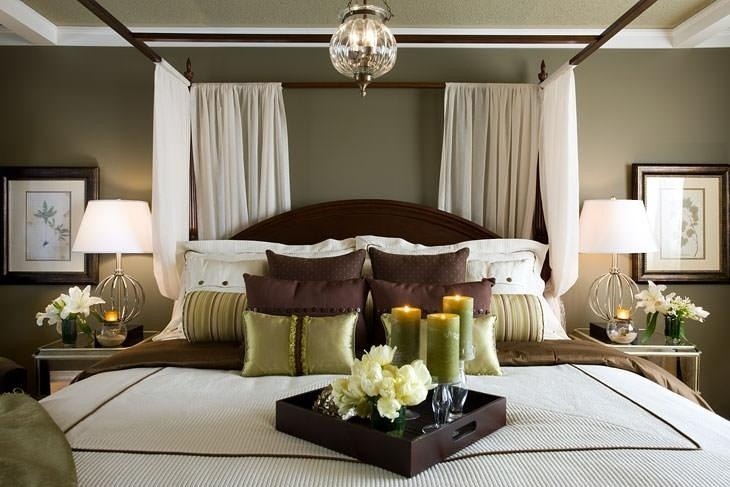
[443,294,473,361]
[427,314,460,381]
[391,305,420,365]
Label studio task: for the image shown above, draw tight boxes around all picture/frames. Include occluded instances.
[634,164,730,285]
[2,166,99,286]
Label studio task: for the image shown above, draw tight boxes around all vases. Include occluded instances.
[61,315,77,345]
[665,317,683,344]
[376,405,406,437]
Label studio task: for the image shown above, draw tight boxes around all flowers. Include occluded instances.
[35,285,105,327]
[332,344,431,420]
[636,280,710,323]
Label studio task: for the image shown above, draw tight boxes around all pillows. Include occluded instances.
[355,235,571,340]
[379,314,501,376]
[265,249,366,282]
[241,304,361,376]
[153,237,354,341]
[368,247,470,283]
[370,277,497,333]
[242,273,369,361]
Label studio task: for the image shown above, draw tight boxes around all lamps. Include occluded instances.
[328,0,399,97]
[576,193,658,317]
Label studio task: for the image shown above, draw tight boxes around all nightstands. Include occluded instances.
[32,331,160,401]
[569,328,702,393]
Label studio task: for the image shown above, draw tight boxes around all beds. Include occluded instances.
[38,198,729,487]
[71,198,155,322]
